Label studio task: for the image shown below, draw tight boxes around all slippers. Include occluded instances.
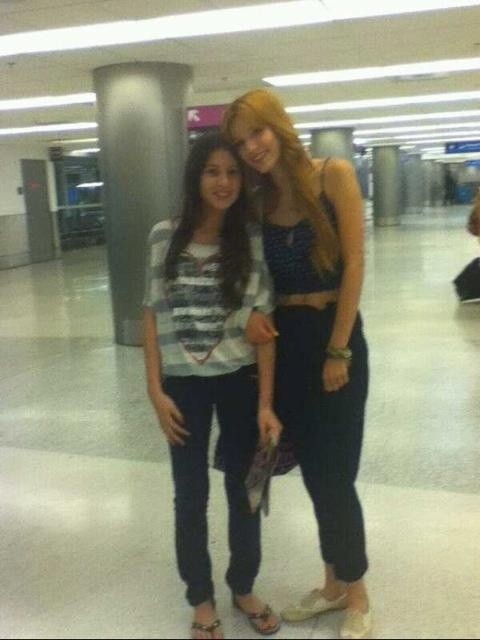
[192,619,223,640]
[231,597,281,635]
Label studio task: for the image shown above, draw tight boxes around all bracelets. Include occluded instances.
[326,345,353,360]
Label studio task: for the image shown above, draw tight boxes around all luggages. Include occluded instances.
[452,256,480,302]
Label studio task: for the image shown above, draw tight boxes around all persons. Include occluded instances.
[443,170,455,206]
[221,88,374,639]
[141,132,282,639]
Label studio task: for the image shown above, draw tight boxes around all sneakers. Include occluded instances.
[281,588,371,640]
